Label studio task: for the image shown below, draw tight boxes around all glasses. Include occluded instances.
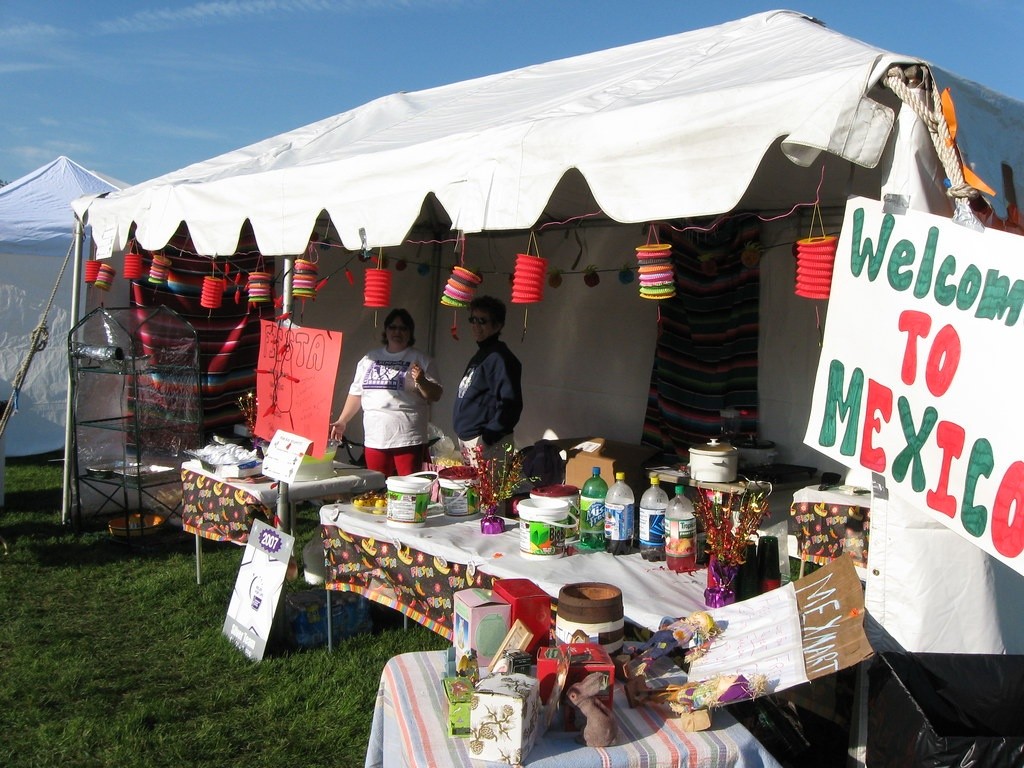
[387,324,410,332]
[468,316,494,325]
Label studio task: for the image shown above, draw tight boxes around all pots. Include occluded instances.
[689,439,739,483]
[732,439,779,469]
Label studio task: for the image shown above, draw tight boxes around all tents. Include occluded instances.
[0,156,135,456]
[62,9,1024,768]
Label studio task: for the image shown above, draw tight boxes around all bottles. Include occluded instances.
[605,473,635,554]
[639,477,669,562]
[578,467,609,552]
[665,484,697,572]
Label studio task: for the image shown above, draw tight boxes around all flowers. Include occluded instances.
[441,443,542,510]
[691,475,771,566]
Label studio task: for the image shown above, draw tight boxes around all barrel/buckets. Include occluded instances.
[437,466,482,517]
[386,470,438,530]
[529,484,579,543]
[516,498,578,556]
[266,586,375,651]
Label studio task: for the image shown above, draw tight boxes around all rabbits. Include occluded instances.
[564,672,618,748]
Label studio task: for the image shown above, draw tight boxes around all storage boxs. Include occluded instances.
[553,435,664,490]
[441,648,539,765]
[536,643,615,732]
[454,578,553,666]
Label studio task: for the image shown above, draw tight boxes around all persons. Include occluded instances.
[453,295,523,484]
[330,309,443,476]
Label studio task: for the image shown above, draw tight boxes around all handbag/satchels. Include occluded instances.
[504,437,572,497]
[302,524,329,585]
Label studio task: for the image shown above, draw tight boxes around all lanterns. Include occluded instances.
[363,268,392,328]
[148,255,171,293]
[248,271,272,302]
[85,260,116,292]
[200,276,223,318]
[511,254,549,344]
[636,244,677,322]
[123,252,142,279]
[795,236,837,347]
[292,258,319,314]
[440,266,481,341]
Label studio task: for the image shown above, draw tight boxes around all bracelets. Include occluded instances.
[415,378,428,388]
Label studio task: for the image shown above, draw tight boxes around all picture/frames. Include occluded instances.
[487,619,534,674]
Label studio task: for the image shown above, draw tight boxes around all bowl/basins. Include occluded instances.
[107,514,166,538]
[257,438,339,481]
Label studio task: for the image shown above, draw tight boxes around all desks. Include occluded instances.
[649,468,821,495]
[364,641,784,768]
[320,503,718,654]
[791,482,871,579]
[181,460,386,585]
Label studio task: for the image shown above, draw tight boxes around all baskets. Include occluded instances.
[98,349,151,370]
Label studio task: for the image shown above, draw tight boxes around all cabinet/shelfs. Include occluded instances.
[66,302,206,562]
